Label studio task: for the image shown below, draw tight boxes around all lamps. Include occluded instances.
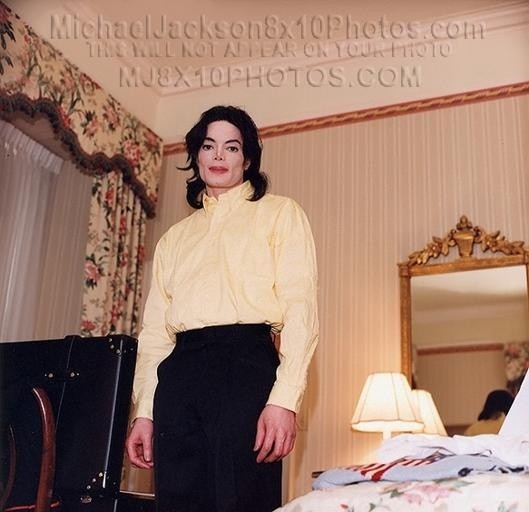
[412,390,448,440]
[350,371,425,439]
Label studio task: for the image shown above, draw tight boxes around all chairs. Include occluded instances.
[0,385,63,511]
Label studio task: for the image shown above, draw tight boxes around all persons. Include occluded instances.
[463,389,515,438]
[125,106,319,510]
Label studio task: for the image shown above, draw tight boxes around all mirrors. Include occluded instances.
[396,214,529,437]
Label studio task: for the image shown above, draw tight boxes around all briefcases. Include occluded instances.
[0,335,138,504]
